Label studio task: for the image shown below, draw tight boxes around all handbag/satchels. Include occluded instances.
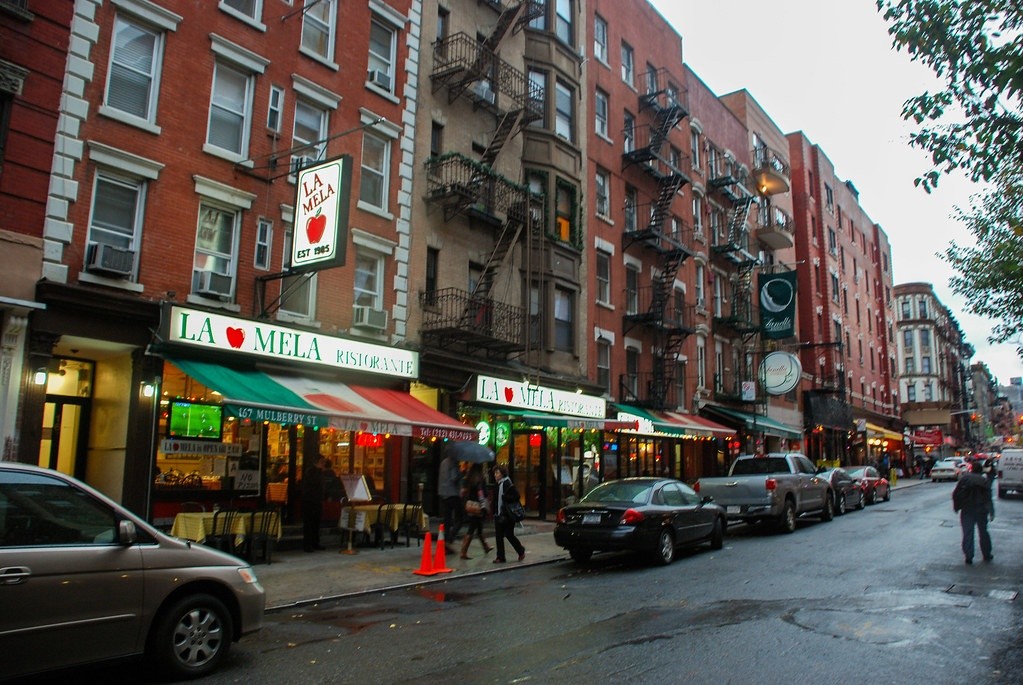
[465,500,483,517]
[504,484,525,523]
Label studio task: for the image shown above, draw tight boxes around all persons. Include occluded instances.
[438,457,463,554]
[952,461,995,565]
[300,453,324,551]
[491,465,526,562]
[461,462,494,559]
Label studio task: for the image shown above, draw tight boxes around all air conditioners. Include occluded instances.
[86,242,138,277]
[368,69,391,91]
[480,87,496,104]
[192,270,235,298]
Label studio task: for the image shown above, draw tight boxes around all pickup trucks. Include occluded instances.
[695,452,835,535]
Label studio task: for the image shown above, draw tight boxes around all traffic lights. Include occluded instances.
[1019,415,1023,423]
[925,446,931,452]
[972,414,981,424]
[1007,438,1012,443]
[956,452,960,456]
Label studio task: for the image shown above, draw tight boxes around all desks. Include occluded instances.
[266,482,287,523]
[337,504,430,549]
[155,475,222,490]
[170,512,283,563]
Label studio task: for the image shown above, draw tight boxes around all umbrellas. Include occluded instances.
[445,439,495,465]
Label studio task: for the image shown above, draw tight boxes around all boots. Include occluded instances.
[460,534,473,560]
[478,534,494,553]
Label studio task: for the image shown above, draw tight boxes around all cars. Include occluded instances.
[817,467,867,514]
[554,478,726,565]
[0,461,266,685]
[841,466,891,506]
[931,462,960,483]
[943,456,969,477]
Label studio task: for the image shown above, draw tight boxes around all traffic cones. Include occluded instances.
[412,523,455,577]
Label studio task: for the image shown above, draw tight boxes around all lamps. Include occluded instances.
[48,358,66,376]
[521,373,530,385]
[762,186,766,191]
[575,387,583,394]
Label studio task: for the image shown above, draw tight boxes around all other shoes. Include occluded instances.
[494,557,505,563]
[445,542,457,553]
[313,542,325,550]
[983,553,993,560]
[519,552,525,561]
[301,544,314,552]
[966,559,972,564]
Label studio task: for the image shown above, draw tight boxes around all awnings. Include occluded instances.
[612,403,737,439]
[490,408,639,430]
[159,349,479,441]
[853,418,902,441]
[704,405,801,440]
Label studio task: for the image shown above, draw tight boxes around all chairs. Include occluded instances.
[360,503,394,551]
[181,474,203,489]
[179,502,205,514]
[394,500,421,548]
[237,506,279,566]
[202,505,240,556]
[341,497,372,548]
[159,474,180,487]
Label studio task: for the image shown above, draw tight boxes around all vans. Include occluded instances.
[997,449,1023,497]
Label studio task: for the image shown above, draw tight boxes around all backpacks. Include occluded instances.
[957,479,975,505]
